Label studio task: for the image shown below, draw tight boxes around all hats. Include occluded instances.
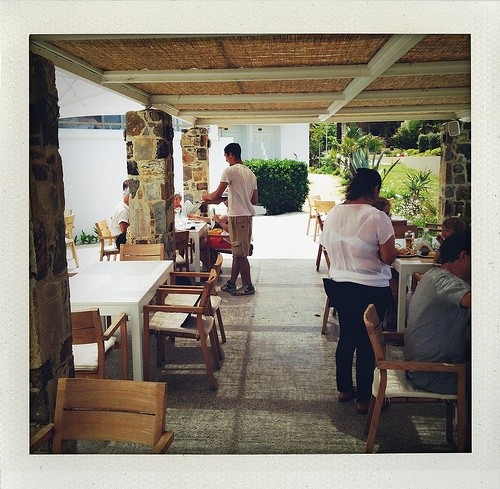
[221,189,228,198]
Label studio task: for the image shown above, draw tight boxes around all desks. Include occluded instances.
[173,217,207,282]
[390,237,443,332]
[69,260,174,382]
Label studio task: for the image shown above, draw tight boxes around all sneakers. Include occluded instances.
[232,285,255,296]
[221,280,236,294]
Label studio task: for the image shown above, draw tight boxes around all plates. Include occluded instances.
[417,251,436,257]
[397,254,416,257]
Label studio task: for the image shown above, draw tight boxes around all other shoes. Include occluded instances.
[338,386,356,402]
[356,397,390,413]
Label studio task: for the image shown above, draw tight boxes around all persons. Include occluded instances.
[123,180,128,190]
[174,191,182,214]
[370,196,409,346]
[110,187,149,256]
[67,116,102,129]
[202,142,259,296]
[318,168,396,416]
[187,188,231,281]
[432,217,466,264]
[403,231,471,453]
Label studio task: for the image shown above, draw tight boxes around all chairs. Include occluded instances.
[308,194,472,454]
[28,209,254,456]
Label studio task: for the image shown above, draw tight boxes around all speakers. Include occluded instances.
[448,121,461,136]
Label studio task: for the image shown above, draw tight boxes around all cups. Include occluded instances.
[405,232,415,250]
